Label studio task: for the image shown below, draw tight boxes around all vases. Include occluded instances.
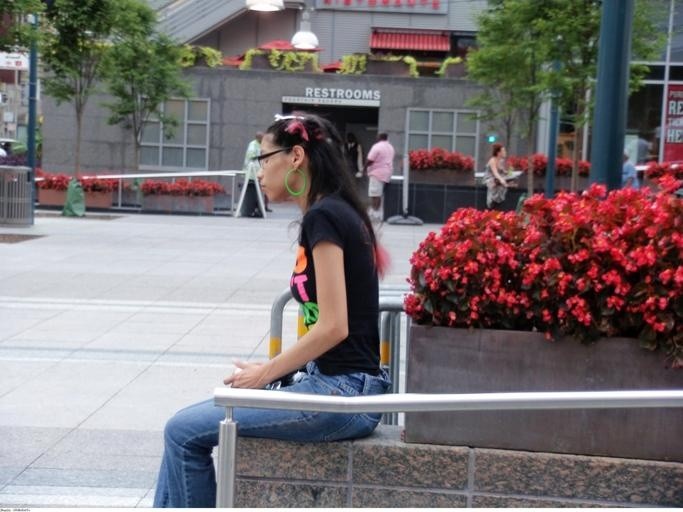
[508,172,586,191]
[408,169,474,185]
[143,193,213,213]
[400,309,683,461]
[37,188,114,209]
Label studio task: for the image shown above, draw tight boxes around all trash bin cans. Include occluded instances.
[0,165,33,225]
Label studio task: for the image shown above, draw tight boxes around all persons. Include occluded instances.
[147,110,394,506]
[242,131,273,212]
[362,132,395,224]
[481,142,519,209]
[620,147,640,191]
[338,132,364,185]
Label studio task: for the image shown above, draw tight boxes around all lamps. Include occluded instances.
[291,0,319,50]
[246,0,284,12]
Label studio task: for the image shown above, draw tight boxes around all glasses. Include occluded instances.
[257,147,292,170]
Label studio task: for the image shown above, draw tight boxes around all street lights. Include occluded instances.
[25,9,45,205]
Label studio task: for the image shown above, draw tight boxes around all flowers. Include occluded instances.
[506,151,592,177]
[409,146,475,170]
[140,178,224,196]
[400,159,683,373]
[33,167,125,194]
